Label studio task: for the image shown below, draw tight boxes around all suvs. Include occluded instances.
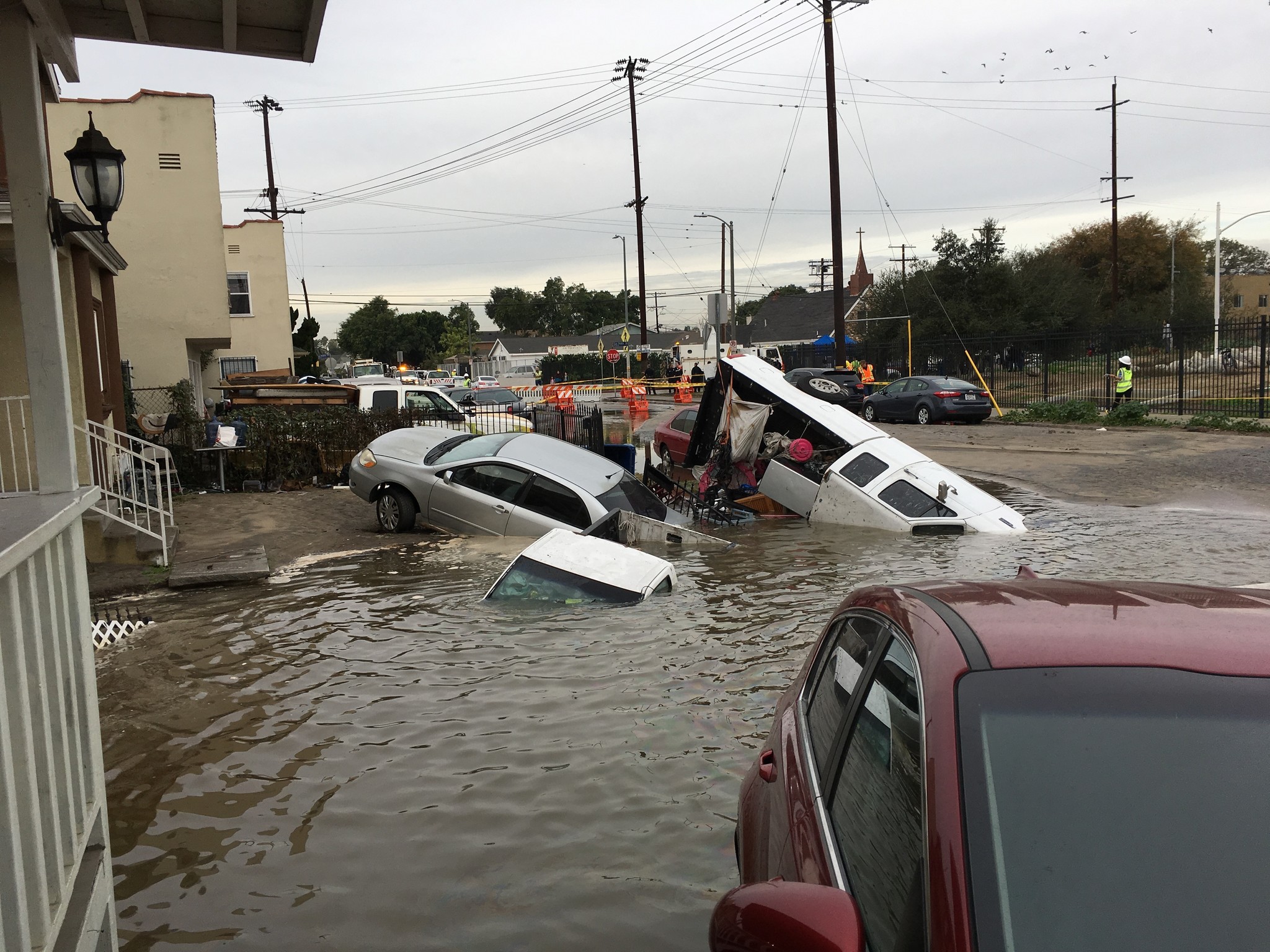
[783,368,866,415]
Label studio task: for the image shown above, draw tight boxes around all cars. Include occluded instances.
[862,376,994,425]
[483,528,681,612]
[384,365,532,421]
[349,428,698,545]
[712,564,1269,952]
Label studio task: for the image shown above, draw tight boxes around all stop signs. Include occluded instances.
[606,349,620,363]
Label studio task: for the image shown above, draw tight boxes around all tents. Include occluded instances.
[814,334,858,345]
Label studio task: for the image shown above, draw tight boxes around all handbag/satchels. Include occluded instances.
[213,425,239,447]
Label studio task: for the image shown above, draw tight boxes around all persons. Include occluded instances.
[550,376,555,385]
[1103,355,1133,407]
[463,373,472,388]
[851,356,861,374]
[563,372,569,383]
[1087,338,1095,356]
[666,362,675,394]
[644,364,657,395]
[555,370,563,384]
[858,359,875,384]
[533,367,542,386]
[1161,319,1172,354]
[673,363,683,394]
[690,363,702,393]
[1003,341,1024,372]
[451,369,458,377]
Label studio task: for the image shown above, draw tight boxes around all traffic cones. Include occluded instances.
[628,385,649,411]
[680,375,695,394]
[674,380,693,403]
[555,389,575,411]
[620,378,636,399]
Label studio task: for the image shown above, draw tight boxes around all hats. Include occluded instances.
[535,367,539,370]
[453,369,456,371]
[860,359,865,365]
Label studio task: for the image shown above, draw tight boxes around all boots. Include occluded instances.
[653,390,656,395]
[649,390,651,395]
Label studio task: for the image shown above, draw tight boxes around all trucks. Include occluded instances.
[339,358,386,380]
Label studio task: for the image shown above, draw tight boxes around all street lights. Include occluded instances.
[612,234,631,381]
[694,214,736,353]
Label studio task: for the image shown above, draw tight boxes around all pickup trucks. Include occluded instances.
[684,353,1037,538]
[245,380,535,482]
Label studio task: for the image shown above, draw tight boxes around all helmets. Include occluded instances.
[464,373,469,377]
[1119,356,1131,365]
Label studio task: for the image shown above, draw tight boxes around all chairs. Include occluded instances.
[140,446,183,493]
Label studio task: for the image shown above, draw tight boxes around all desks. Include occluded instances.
[194,445,247,493]
